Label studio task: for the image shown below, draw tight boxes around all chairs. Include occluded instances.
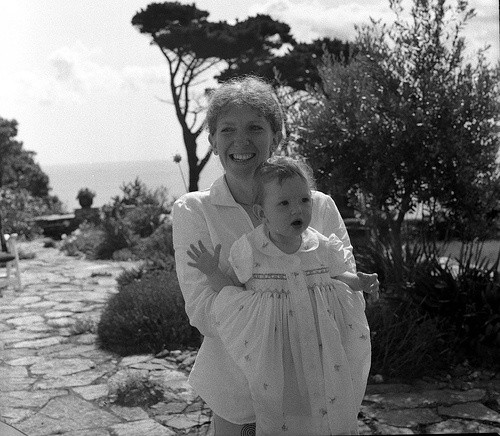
[0,230,21,294]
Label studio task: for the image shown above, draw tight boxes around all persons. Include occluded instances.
[170,76,360,435]
[186,156,383,436]
